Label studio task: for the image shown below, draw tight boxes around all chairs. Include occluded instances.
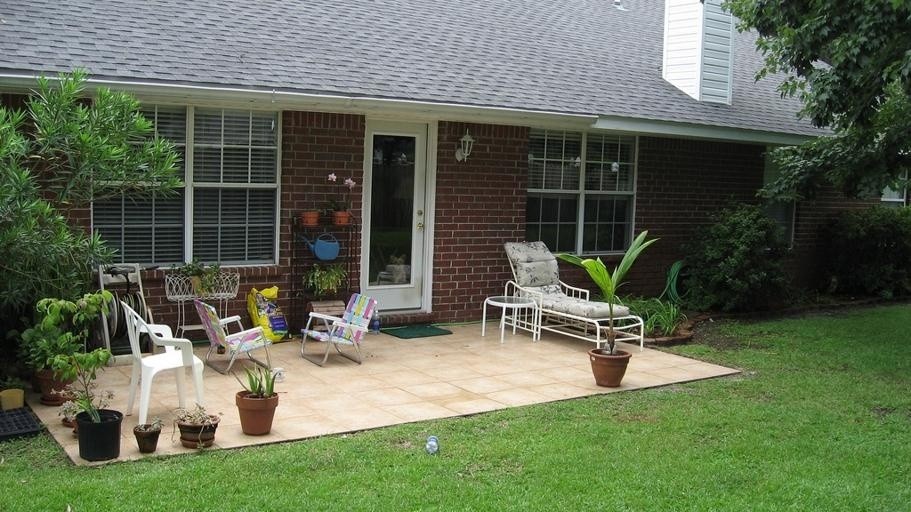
[376,265,411,285]
[301,293,378,366]
[117,300,205,426]
[504,241,645,353]
[194,299,273,374]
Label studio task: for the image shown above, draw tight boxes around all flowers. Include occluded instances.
[327,173,356,211]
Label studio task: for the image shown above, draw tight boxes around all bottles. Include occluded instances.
[426,435,440,454]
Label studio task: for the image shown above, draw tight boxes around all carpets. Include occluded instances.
[380,325,453,339]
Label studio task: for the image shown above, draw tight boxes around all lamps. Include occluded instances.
[455,127,475,162]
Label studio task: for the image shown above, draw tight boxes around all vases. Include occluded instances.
[0,388,24,411]
[301,211,320,226]
[332,210,350,225]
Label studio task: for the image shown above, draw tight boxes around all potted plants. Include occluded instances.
[13,288,124,461]
[133,416,166,453]
[231,362,280,435]
[302,260,348,296]
[554,229,661,388]
[171,403,221,453]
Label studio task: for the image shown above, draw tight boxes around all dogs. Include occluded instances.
[389,254,407,284]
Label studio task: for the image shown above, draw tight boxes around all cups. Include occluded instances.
[2,388,26,412]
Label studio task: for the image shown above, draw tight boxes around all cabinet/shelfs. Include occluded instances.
[289,216,361,340]
[165,272,240,350]
